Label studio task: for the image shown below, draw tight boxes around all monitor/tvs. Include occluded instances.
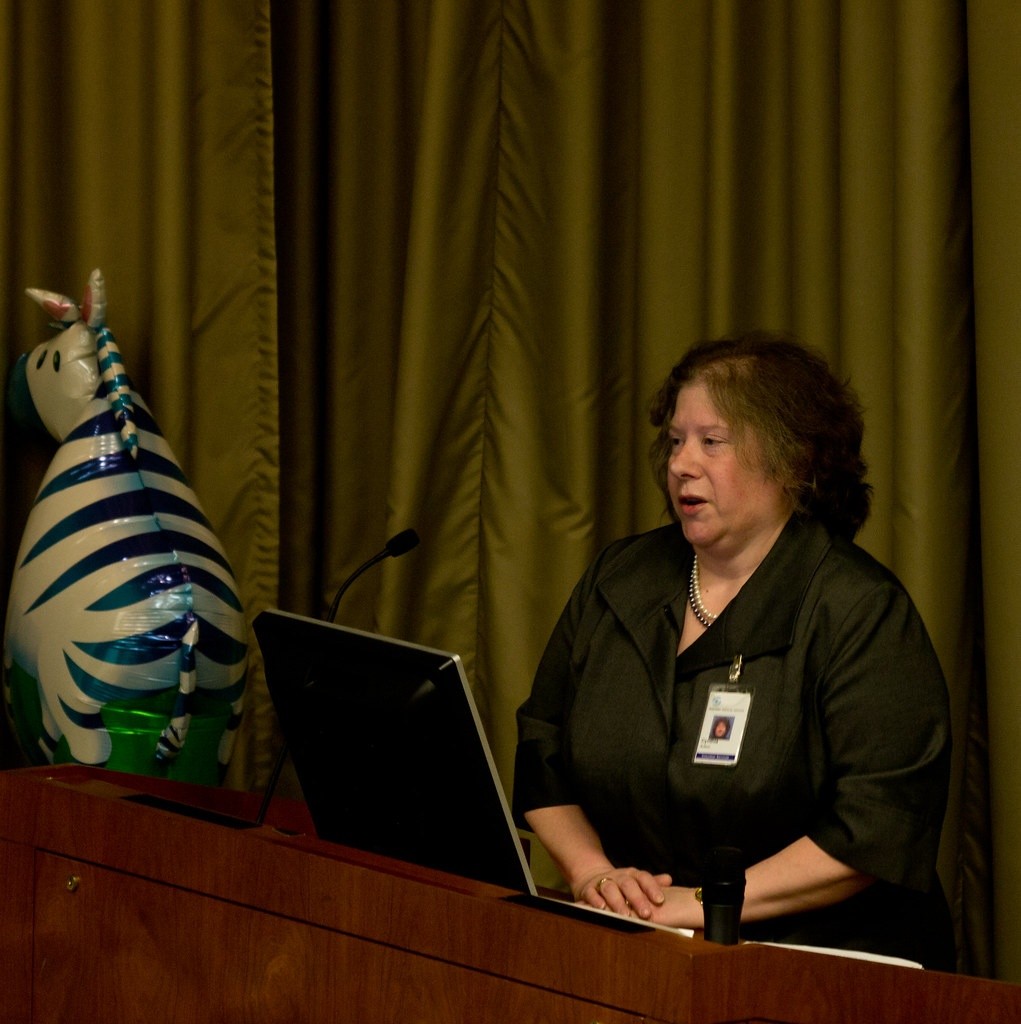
[255,608,539,901]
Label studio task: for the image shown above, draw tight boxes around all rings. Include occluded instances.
[598,877,613,893]
[626,900,633,916]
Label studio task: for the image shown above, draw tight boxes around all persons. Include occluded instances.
[711,717,730,738]
[513,331,958,971]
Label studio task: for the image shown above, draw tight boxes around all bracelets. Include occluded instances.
[695,888,703,904]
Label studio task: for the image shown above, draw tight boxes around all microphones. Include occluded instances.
[257,530,421,826]
[701,846,746,947]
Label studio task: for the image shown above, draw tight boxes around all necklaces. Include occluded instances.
[689,555,717,626]
